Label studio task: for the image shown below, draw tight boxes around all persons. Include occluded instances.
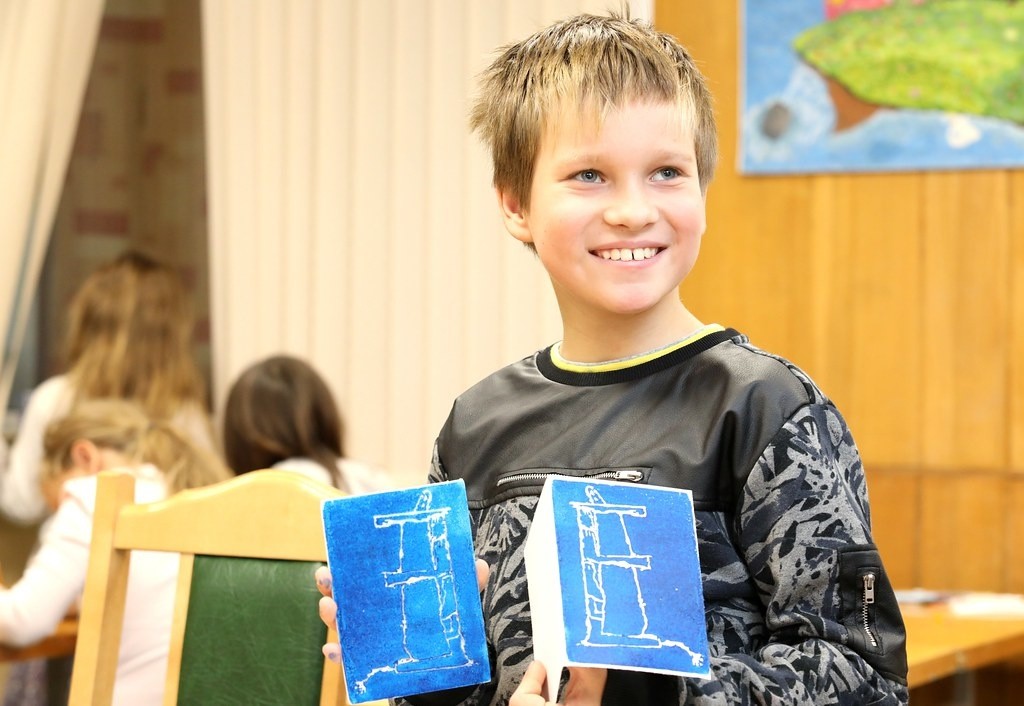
[222,355,389,502]
[0,259,233,706]
[315,0,911,706]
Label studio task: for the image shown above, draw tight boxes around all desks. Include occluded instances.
[898,594,1024,706]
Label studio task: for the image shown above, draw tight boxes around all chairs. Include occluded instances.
[68,466,352,706]
